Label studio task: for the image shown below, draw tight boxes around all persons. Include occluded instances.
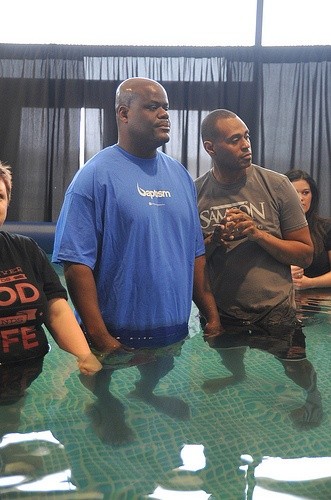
[284,168,331,292]
[192,108,325,360]
[52,78,225,368]
[0,164,102,407]
